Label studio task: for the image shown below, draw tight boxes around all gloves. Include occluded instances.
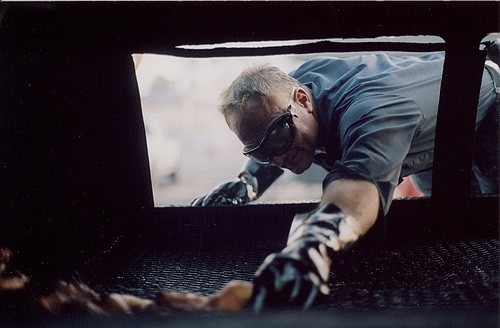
[192,157,285,207]
[247,204,362,313]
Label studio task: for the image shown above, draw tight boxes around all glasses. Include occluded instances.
[243,87,301,163]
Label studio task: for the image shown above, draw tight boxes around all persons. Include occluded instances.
[191,39,500,313]
